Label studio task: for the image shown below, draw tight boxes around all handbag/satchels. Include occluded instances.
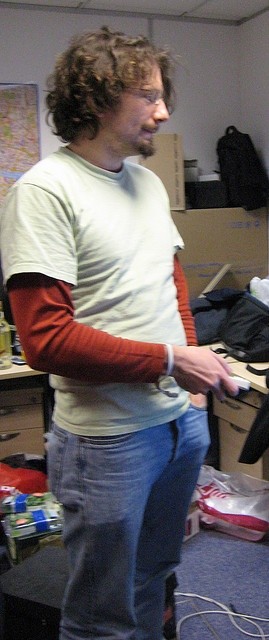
[187,287,239,345]
[217,290,268,363]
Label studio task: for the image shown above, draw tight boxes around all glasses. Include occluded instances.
[122,84,165,103]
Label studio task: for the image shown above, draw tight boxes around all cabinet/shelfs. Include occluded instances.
[0,388,54,460]
[211,391,269,481]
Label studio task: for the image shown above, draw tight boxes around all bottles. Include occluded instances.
[0,312,11,370]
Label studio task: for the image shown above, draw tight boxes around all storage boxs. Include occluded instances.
[1,541,179,639]
[171,208,269,301]
[140,134,186,209]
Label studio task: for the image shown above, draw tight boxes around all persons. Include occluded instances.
[8,29,240,635]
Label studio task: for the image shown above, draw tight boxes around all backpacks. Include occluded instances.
[216,126,269,211]
